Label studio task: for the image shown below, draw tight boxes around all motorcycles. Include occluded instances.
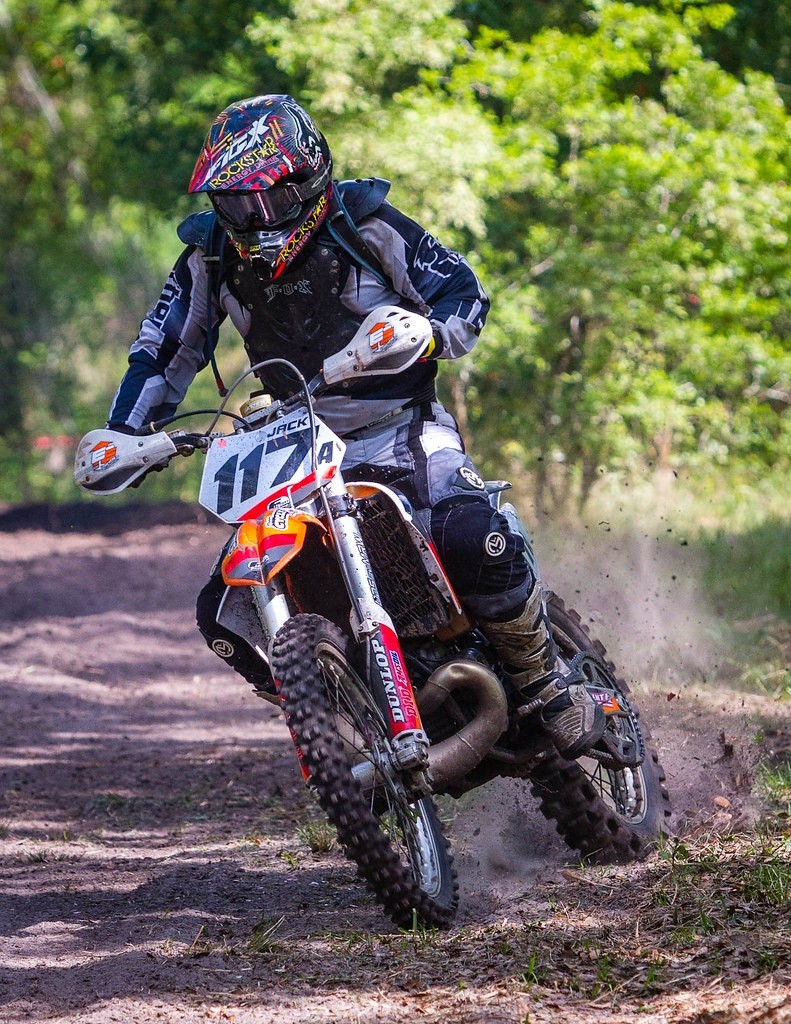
[72,307,670,929]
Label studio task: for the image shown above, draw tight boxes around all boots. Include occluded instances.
[482,580,607,762]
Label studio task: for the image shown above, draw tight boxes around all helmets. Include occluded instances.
[186,93,332,281]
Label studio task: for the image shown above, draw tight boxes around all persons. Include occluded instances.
[101,95,606,760]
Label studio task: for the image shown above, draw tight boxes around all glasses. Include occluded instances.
[208,182,309,229]
